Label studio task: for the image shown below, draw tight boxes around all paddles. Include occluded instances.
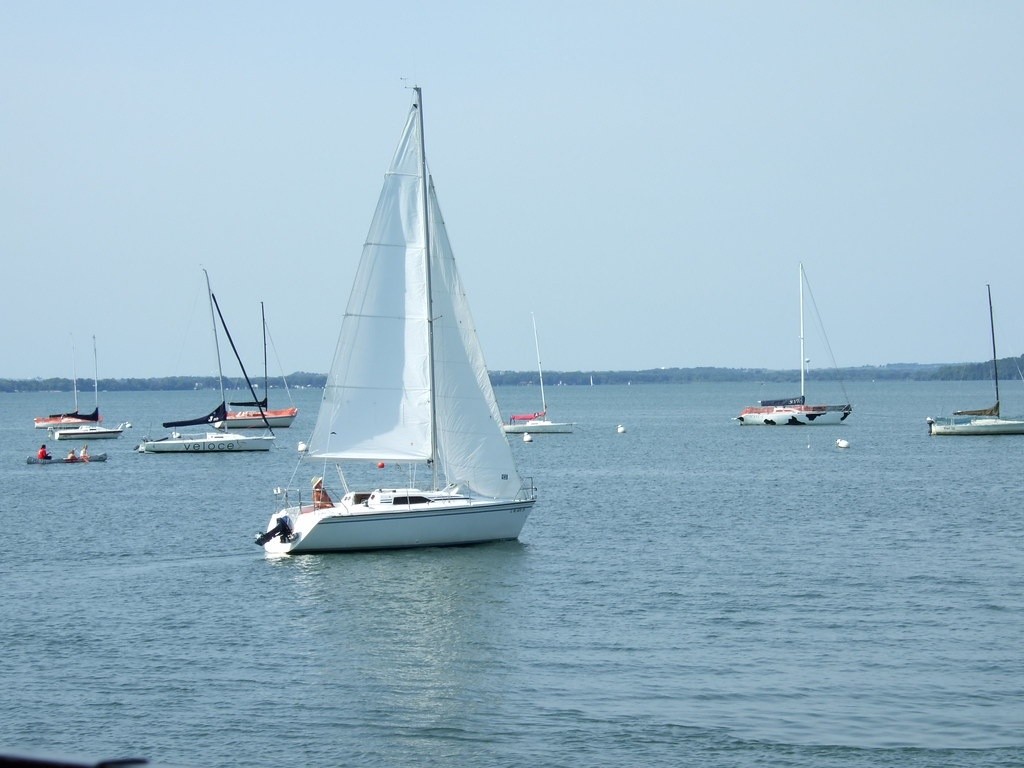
[73,448,89,463]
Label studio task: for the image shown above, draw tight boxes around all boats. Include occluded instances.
[27,451,107,465]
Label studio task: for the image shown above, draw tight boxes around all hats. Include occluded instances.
[310,476,322,489]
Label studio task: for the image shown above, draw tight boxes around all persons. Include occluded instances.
[38,445,52,460]
[311,475,335,510]
[68,450,78,459]
[80,444,90,464]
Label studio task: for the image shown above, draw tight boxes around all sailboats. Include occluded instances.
[135,268,277,453]
[33,332,104,430]
[254,74,538,554]
[732,259,853,427]
[501,311,574,434]
[47,332,134,440]
[214,300,299,428]
[926,283,1024,436]
